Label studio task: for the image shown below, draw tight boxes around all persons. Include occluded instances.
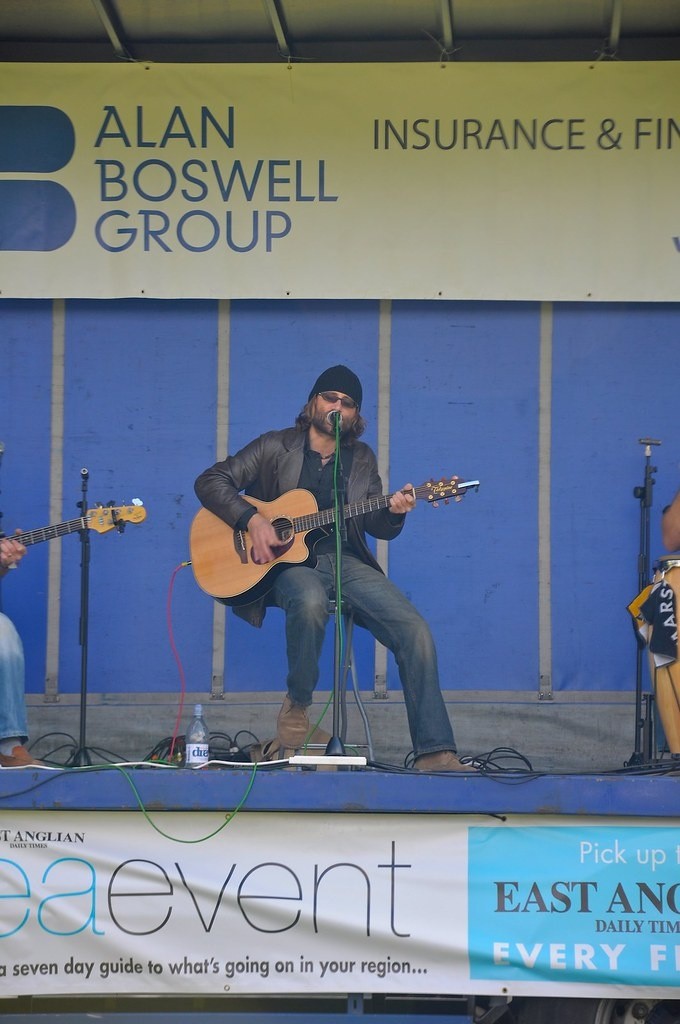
[0,442,48,768]
[194,363,479,774]
[658,489,680,554]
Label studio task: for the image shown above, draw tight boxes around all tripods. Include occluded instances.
[304,422,419,772]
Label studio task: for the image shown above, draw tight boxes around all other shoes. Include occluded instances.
[0,746,44,767]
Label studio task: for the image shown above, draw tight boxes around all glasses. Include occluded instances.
[317,392,356,408]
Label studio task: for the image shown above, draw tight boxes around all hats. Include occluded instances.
[308,365,362,413]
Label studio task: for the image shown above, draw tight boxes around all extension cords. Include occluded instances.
[289,755,367,766]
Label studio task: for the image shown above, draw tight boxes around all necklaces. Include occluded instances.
[319,448,336,459]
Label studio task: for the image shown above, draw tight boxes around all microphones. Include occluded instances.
[327,411,343,426]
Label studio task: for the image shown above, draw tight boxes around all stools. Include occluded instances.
[279,600,374,769]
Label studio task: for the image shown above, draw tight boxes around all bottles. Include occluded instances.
[186,704,210,768]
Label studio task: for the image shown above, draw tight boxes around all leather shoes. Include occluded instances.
[277,693,309,748]
[412,752,476,773]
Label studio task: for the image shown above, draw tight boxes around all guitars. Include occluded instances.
[2,501,148,549]
[190,474,479,607]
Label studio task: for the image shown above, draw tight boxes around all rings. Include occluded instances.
[7,554,12,557]
